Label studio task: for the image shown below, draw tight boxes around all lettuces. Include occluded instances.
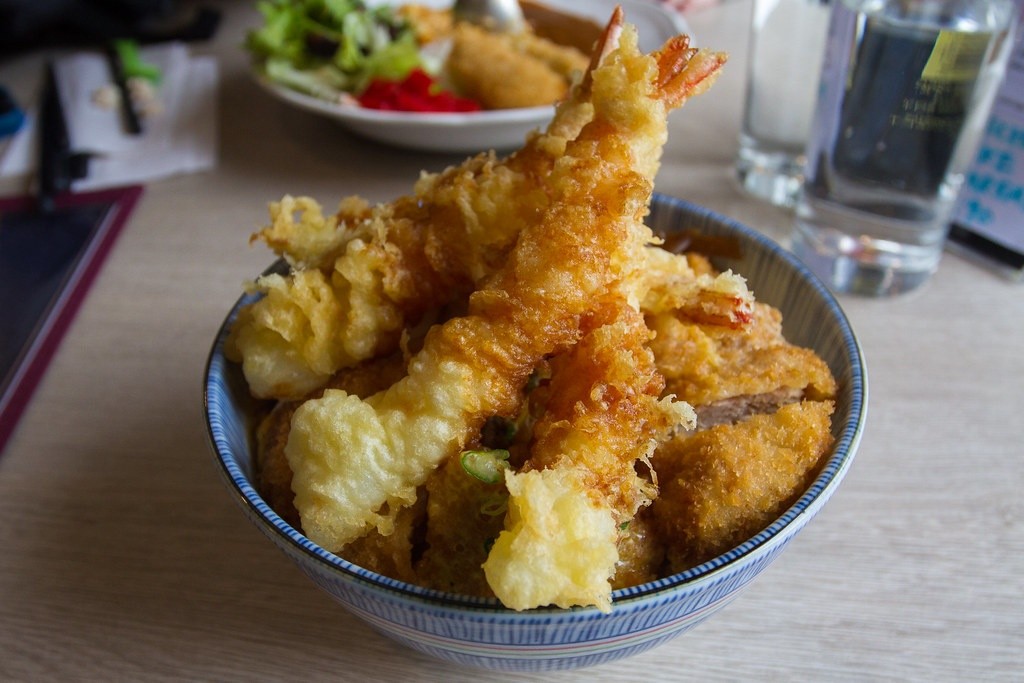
[239,2,442,109]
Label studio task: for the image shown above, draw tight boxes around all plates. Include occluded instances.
[242,0,695,156]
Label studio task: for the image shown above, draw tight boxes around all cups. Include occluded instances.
[735,0,883,216]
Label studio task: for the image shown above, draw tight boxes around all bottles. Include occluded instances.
[791,0,1019,299]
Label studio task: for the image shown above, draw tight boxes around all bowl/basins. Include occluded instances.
[200,173,871,672]
